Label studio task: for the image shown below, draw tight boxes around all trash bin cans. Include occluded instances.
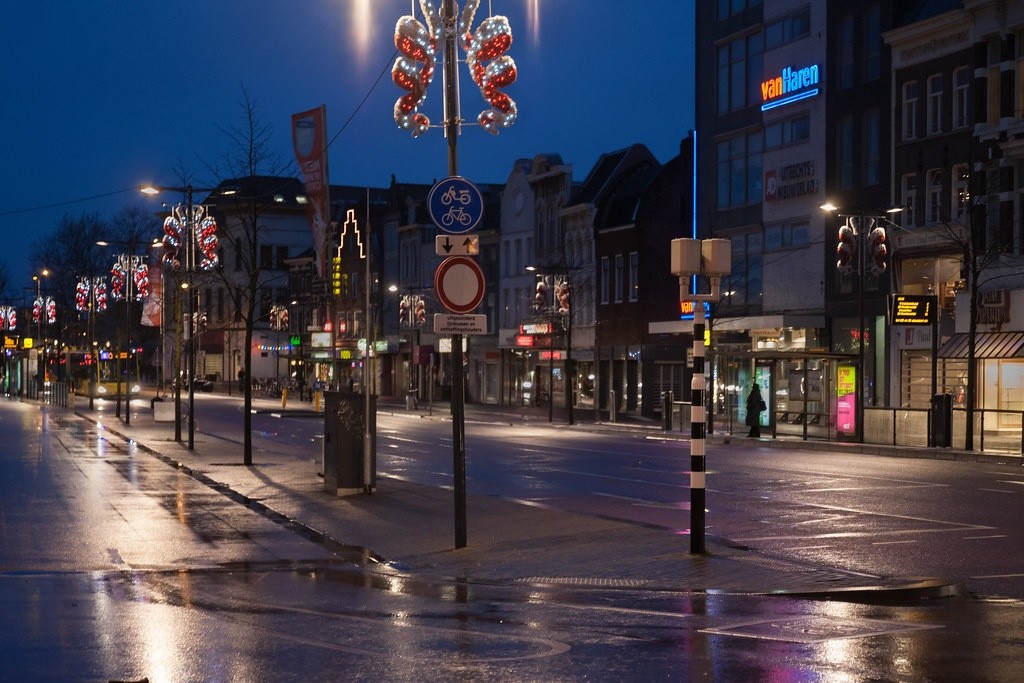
[930,394,957,445]
[322,389,378,487]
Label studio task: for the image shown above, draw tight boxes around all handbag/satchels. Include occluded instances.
[760,401,767,411]
[746,416,751,426]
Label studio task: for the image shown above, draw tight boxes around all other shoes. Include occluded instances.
[747,433,760,437]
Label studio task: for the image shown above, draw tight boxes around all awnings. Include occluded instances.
[936,333,1024,359]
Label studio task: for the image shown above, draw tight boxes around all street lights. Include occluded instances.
[669,237,733,555]
[0,277,108,412]
[387,285,434,412]
[94,239,165,425]
[818,202,906,444]
[136,185,237,451]
[523,265,582,426]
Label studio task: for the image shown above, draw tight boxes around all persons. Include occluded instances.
[745,382,761,440]
[238,366,246,392]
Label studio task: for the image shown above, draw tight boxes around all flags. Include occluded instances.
[290,106,325,280]
[141,265,161,326]
